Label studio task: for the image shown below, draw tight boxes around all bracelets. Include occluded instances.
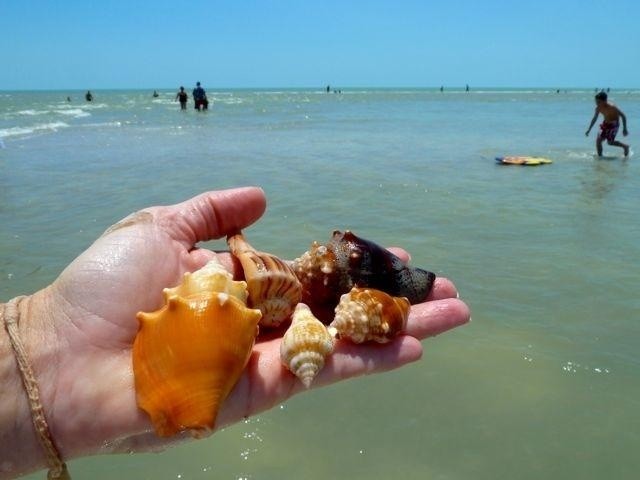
[3,293,72,480]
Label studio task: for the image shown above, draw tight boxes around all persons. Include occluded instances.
[1,186,473,480]
[585,91,631,158]
[174,86,189,111]
[85,89,93,102]
[191,81,210,111]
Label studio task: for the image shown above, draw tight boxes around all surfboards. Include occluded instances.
[495,156,552,166]
